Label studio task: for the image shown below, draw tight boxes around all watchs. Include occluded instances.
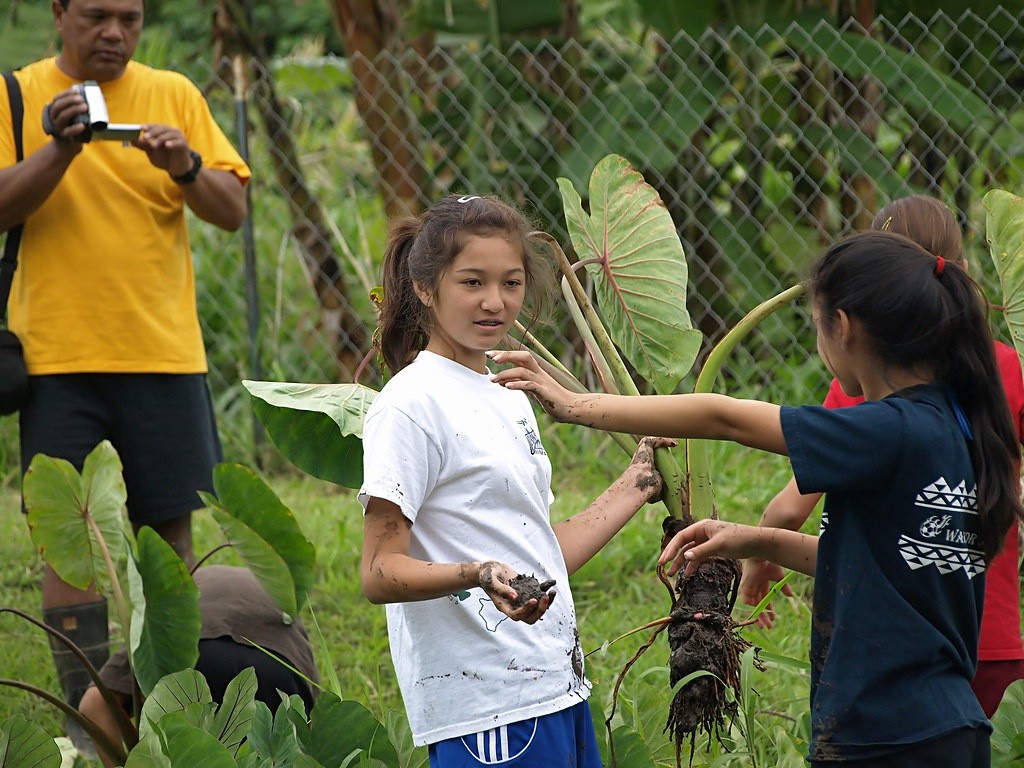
[169,151,201,185]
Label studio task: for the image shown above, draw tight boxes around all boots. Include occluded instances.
[42,598,108,762]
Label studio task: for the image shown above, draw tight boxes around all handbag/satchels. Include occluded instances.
[0,330,34,416]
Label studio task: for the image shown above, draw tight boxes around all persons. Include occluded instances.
[79,565,319,767]
[0,1,251,757]
[739,196,1024,720]
[355,195,678,768]
[483,233,1024,768]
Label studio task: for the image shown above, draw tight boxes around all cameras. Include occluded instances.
[70,80,142,143]
